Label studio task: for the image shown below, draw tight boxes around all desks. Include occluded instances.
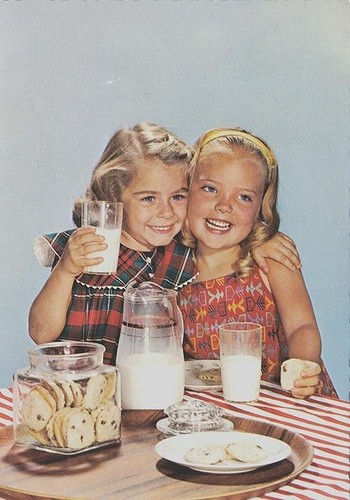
[0,380,349,500]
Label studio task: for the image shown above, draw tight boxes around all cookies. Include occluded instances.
[19,369,121,449]
[197,369,221,382]
[186,440,267,465]
[280,358,309,391]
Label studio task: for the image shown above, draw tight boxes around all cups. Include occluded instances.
[218,322,262,402]
[80,203,121,274]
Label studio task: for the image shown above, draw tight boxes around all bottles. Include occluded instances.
[12,342,122,455]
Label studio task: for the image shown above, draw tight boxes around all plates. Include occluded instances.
[156,418,233,435]
[184,359,222,391]
[156,431,291,474]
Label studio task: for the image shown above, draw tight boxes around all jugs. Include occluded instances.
[115,282,185,412]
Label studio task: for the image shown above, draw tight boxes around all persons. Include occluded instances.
[29,123,303,366]
[174,126,340,400]
